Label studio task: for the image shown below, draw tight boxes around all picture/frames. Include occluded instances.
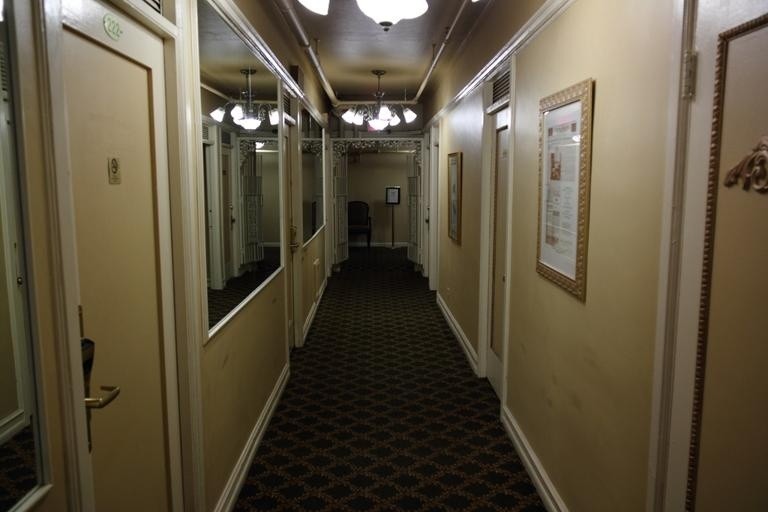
[447,151,462,246]
[535,78,594,306]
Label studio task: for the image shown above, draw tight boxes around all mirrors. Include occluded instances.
[297,100,326,249]
[192,0,286,349]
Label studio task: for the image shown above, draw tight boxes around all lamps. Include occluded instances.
[340,69,419,134]
[208,67,279,132]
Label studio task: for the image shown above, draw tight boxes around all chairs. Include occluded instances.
[347,201,372,250]
